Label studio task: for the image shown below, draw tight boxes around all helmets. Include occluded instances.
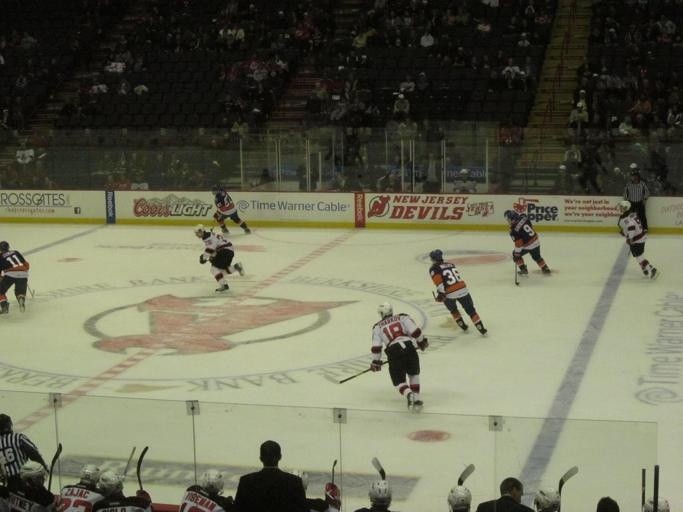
[504,209,519,222]
[19,460,47,486]
[0,241,9,251]
[289,469,309,490]
[643,495,669,512]
[368,479,392,509]
[201,469,224,493]
[80,463,101,489]
[377,302,394,320]
[429,249,444,263]
[620,200,632,213]
[98,469,123,495]
[193,224,206,233]
[534,486,561,511]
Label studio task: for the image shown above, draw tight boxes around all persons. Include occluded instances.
[0,241,30,315]
[210,185,252,236]
[558,0,683,198]
[595,496,621,512]
[369,303,429,414]
[617,205,661,280]
[533,488,561,512]
[353,479,392,512]
[426,249,489,336]
[502,209,554,275]
[0,413,51,487]
[474,477,536,511]
[624,169,651,234]
[193,224,246,293]
[0,1,549,199]
[0,438,344,511]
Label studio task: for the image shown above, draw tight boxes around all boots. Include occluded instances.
[474,320,487,335]
[517,265,528,274]
[540,264,551,274]
[455,317,468,331]
[220,224,228,233]
[241,221,251,234]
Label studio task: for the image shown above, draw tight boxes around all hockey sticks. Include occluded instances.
[458,464,475,486]
[431,291,437,300]
[27,283,35,298]
[558,466,579,512]
[210,219,215,231]
[372,456,385,480]
[48,443,62,489]
[124,446,136,475]
[514,263,520,285]
[137,446,149,490]
[331,460,338,484]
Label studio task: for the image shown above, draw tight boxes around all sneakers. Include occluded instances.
[215,284,230,292]
[234,262,243,277]
[18,297,26,312]
[0,301,9,314]
[407,392,424,415]
[642,265,657,280]
